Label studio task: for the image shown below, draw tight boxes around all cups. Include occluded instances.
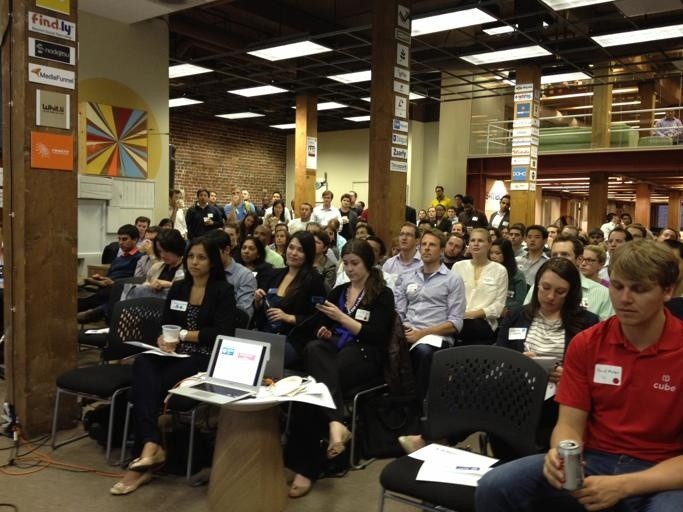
[162,324,181,343]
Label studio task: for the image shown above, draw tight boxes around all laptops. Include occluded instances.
[167,334,271,407]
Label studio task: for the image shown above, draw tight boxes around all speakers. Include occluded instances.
[169,145,176,189]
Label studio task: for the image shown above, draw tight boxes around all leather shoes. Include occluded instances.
[290,484,311,497]
[398,436,417,453]
[327,431,352,459]
[110,446,166,494]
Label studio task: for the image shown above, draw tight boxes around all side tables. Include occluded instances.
[207,402,288,512]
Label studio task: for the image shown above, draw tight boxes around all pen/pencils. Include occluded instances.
[523,342,531,353]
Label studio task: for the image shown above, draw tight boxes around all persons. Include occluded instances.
[652,104,682,144]
[77,187,682,512]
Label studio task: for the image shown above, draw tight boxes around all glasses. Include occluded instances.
[580,256,597,263]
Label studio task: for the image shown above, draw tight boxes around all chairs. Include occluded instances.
[78,277,152,366]
[378,344,550,511]
[51,298,170,457]
[119,308,250,481]
[346,382,389,470]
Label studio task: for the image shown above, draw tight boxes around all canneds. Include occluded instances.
[557,440,583,490]
